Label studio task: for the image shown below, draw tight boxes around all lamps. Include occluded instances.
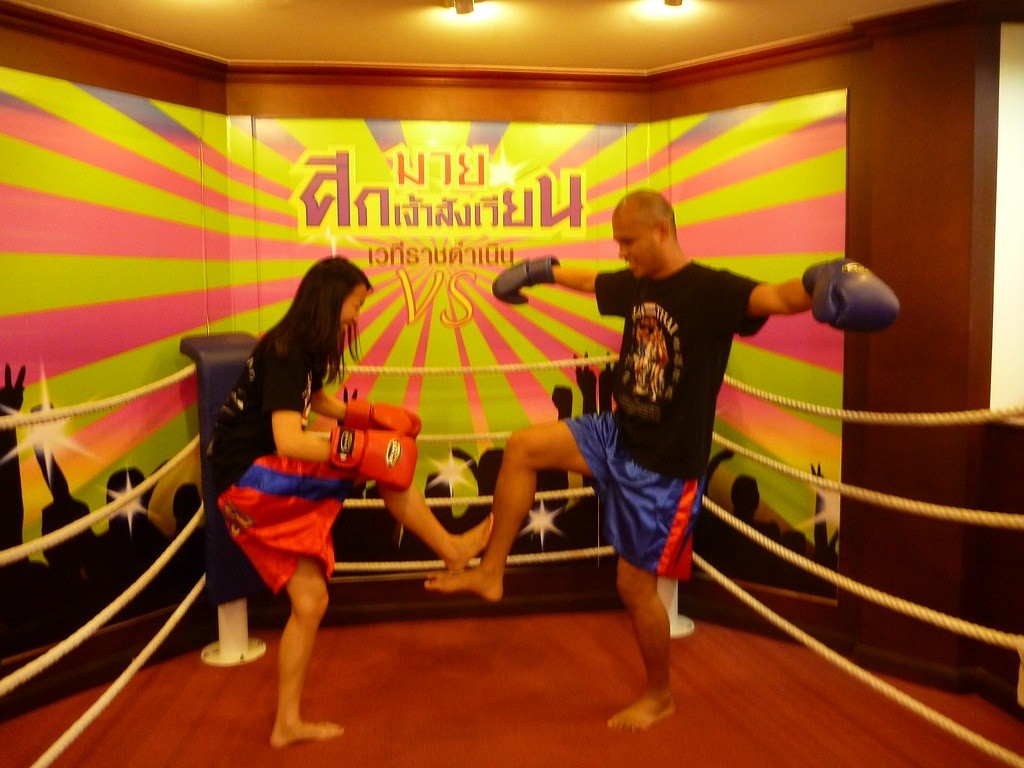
[446,0,484,14]
[665,0,682,6]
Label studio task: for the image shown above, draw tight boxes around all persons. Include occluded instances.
[425,191,899,729]
[206,255,493,746]
